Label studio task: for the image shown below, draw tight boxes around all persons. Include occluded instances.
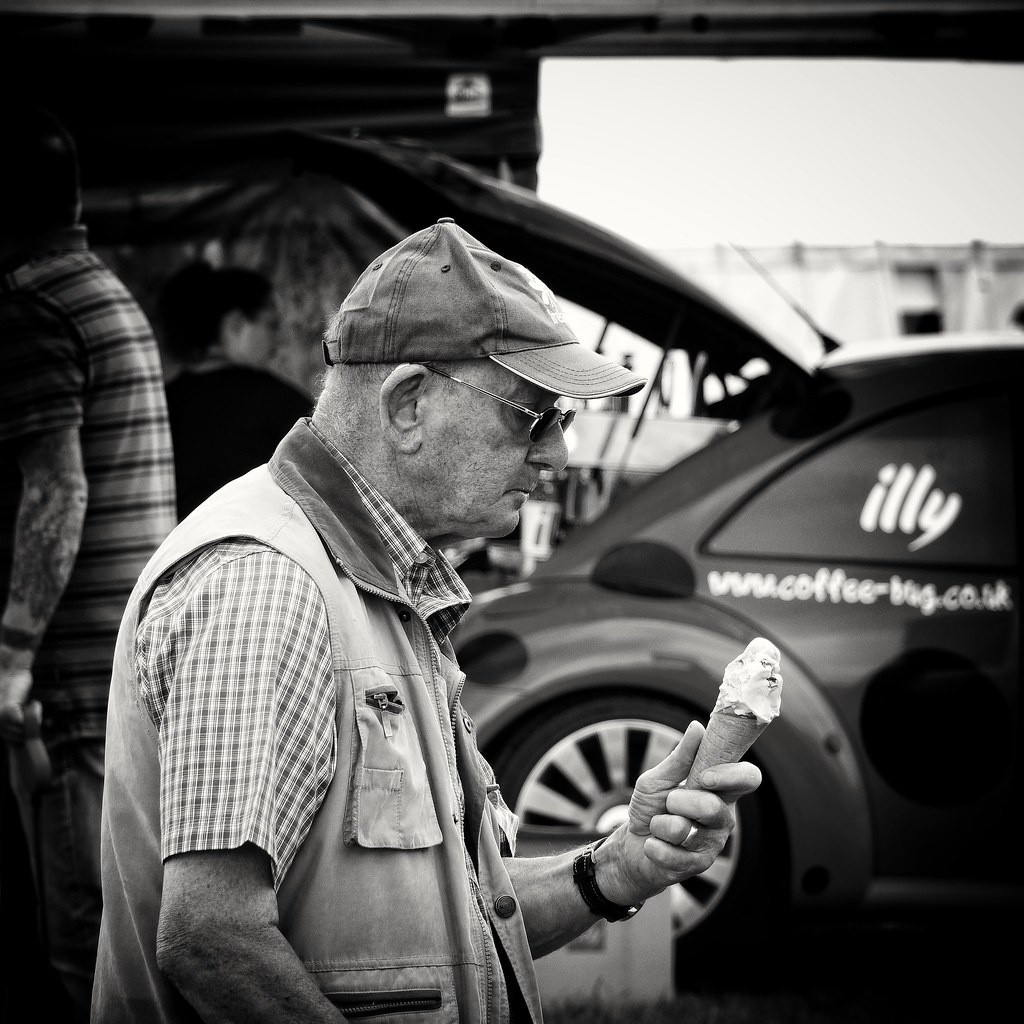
[0,126,315,1024]
[89,217,763,1024]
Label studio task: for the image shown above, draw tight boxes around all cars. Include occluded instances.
[433,344,1022,979]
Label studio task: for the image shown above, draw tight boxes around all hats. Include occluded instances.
[322,215,645,400]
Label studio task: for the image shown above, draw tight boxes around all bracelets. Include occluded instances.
[1,624,44,653]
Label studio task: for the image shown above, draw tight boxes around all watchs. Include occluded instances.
[574,837,646,923]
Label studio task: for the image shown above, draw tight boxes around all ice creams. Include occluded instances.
[683,636,783,792]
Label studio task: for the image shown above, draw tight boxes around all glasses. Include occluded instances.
[424,364,577,443]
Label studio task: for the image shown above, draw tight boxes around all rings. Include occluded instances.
[680,823,699,848]
